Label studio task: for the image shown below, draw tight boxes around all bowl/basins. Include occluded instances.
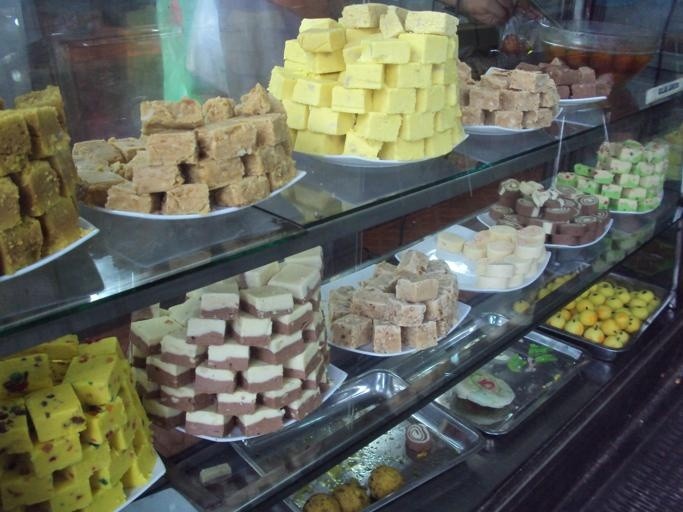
[538,17,660,89]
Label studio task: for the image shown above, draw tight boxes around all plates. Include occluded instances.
[118,488,197,511]
[112,448,166,510]
[318,259,471,358]
[78,164,308,221]
[395,183,667,293]
[464,91,609,137]
[289,129,472,168]
[0,214,98,284]
[167,356,348,446]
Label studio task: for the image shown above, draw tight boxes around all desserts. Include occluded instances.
[1,129,682,511]
[0,3,659,275]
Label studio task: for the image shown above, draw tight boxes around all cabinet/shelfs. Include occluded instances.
[0,73,683,512]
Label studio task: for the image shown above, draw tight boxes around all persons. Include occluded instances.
[184,2,544,108]
